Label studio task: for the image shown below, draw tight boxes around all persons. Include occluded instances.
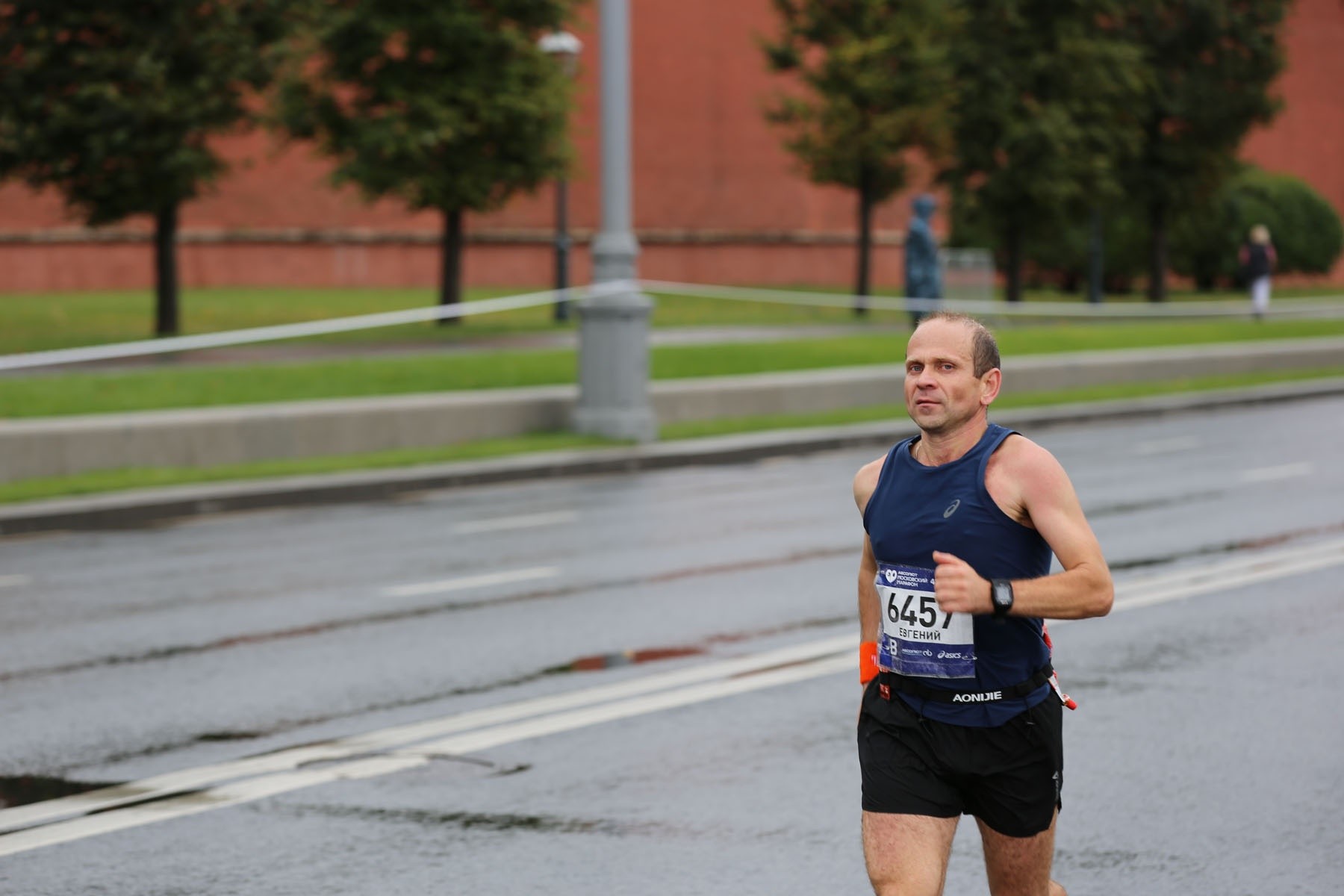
[1238,227,1275,316]
[902,194,945,333]
[853,311,1117,896]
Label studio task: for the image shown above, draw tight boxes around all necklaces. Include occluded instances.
[915,443,923,462]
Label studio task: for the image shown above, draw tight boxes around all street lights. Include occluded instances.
[535,21,586,322]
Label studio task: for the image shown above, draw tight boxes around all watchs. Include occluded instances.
[989,577,1014,614]
